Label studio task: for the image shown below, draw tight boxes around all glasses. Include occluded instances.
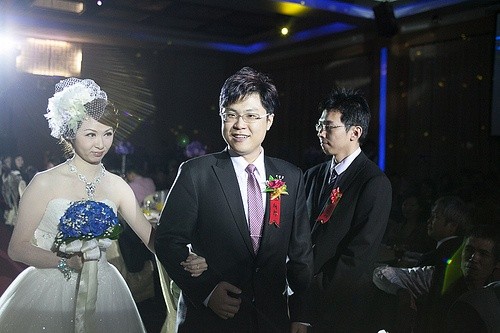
[315,124,351,132]
[220,112,269,123]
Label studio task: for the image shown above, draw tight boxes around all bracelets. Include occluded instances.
[57,258,71,279]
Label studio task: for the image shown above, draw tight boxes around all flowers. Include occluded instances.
[43,83,94,139]
[53,197,123,333]
[329,187,342,203]
[261,175,289,201]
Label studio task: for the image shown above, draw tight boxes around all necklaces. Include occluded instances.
[66,159,105,197]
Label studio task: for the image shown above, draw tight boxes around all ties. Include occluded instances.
[246,164,264,256]
[319,169,338,213]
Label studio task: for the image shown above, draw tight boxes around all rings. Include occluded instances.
[197,264,200,270]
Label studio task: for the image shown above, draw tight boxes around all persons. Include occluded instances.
[155,67,313,333]
[0,147,500,333]
[303,88,391,333]
[0,78,207,333]
